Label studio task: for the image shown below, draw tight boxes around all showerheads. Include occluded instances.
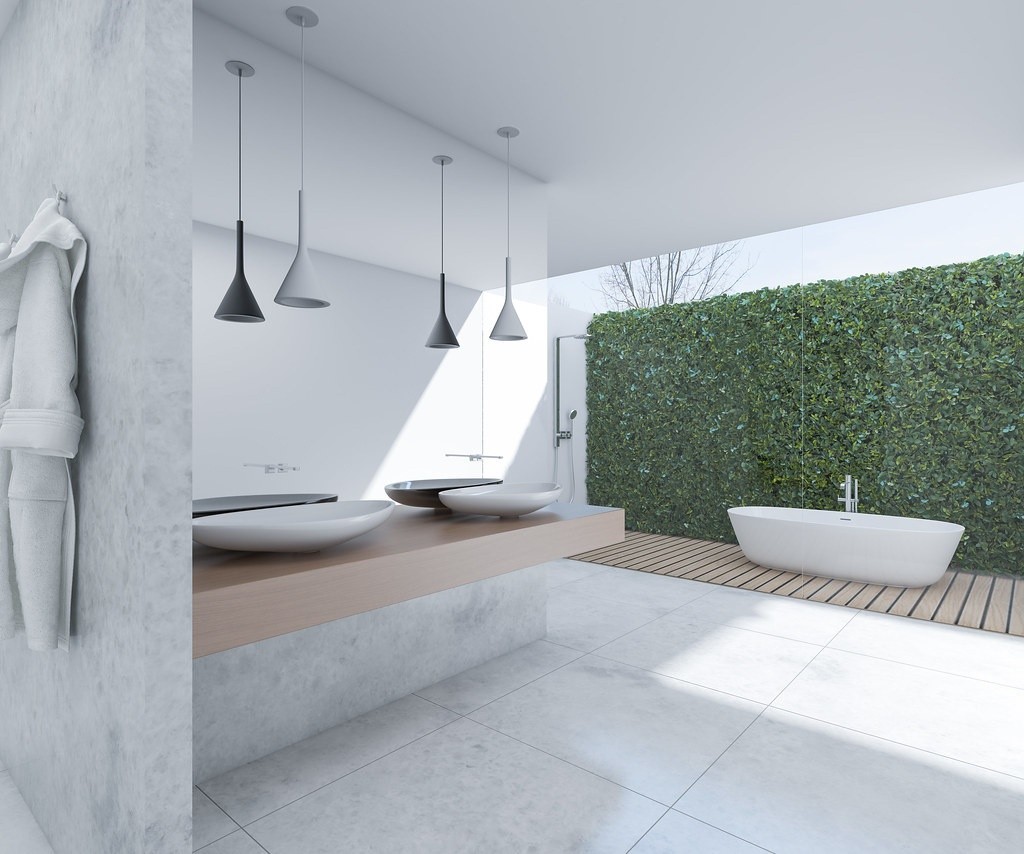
[574,332,594,340]
[569,408,578,439]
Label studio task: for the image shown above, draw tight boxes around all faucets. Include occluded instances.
[839,474,853,512]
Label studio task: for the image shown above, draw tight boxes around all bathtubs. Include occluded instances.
[728,506,966,589]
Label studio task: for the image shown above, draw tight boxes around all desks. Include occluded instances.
[193,495,624,661]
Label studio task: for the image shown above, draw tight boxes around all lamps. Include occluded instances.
[212,59,266,324]
[490,124,529,342]
[273,6,331,308]
[423,154,461,349]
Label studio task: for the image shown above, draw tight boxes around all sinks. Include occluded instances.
[438,481,564,519]
[192,493,338,520]
[191,499,396,553]
[384,478,503,513]
